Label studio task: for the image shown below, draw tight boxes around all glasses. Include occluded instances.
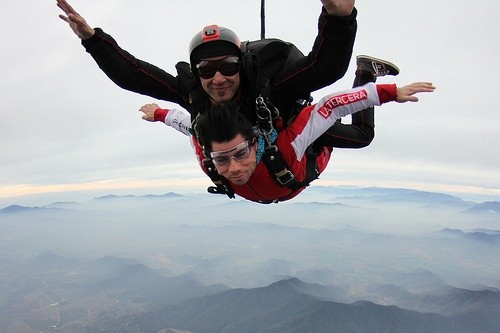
[210,137,256,167]
[196,56,240,79]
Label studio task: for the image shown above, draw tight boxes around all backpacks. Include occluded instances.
[192,39,305,107]
[190,98,319,198]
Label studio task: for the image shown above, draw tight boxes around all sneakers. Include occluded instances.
[356,54,393,77]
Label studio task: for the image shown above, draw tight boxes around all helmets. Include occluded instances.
[189,25,241,74]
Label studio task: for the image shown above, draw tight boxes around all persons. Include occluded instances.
[56,0,436,205]
